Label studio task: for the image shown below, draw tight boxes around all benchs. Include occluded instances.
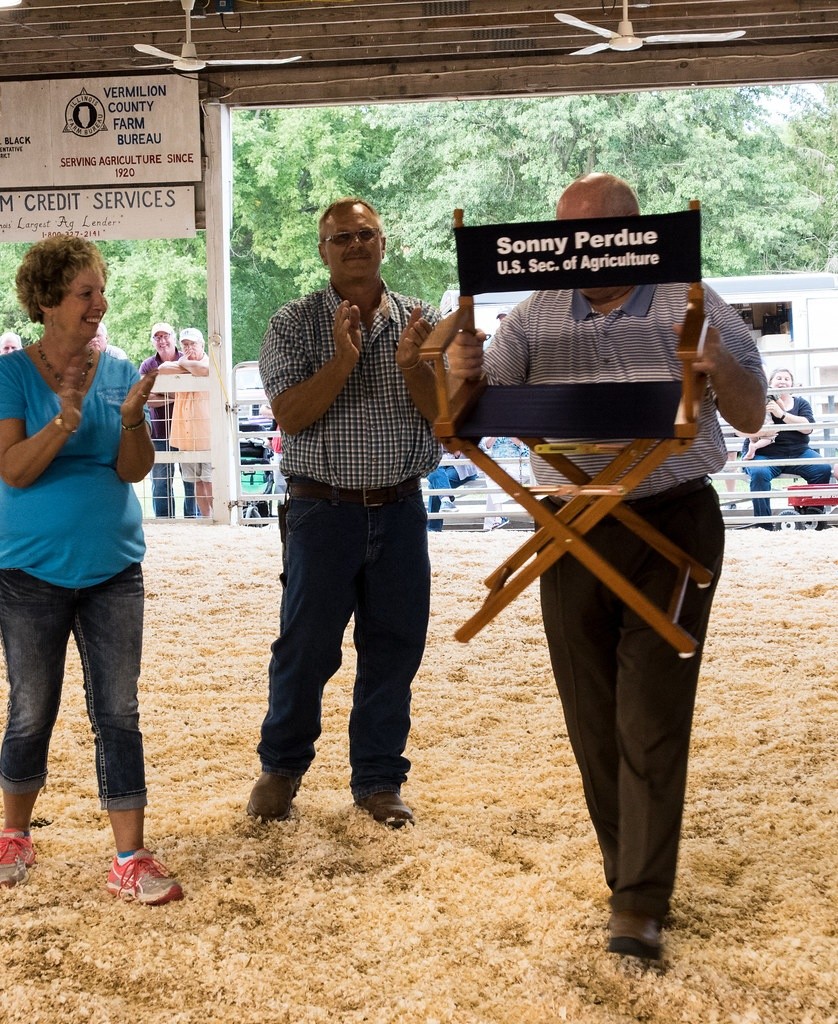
[238,345,838,529]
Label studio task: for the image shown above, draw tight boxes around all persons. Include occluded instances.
[92,322,127,359]
[157,327,213,518]
[246,197,449,828]
[0,235,184,907]
[259,404,289,505]
[139,322,202,519]
[477,436,530,531]
[0,332,23,356]
[427,434,478,532]
[716,367,838,531]
[448,170,767,959]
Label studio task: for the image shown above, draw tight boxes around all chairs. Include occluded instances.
[419,200,713,657]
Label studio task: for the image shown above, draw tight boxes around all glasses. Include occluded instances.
[324,230,378,244]
[153,335,171,340]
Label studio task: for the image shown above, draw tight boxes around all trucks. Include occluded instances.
[440,272,838,439]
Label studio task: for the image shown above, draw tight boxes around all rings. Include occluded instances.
[142,394,146,397]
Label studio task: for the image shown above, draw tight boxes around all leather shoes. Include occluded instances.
[248,772,302,823]
[355,792,415,830]
[610,913,663,960]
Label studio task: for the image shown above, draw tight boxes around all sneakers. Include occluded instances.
[439,501,459,512]
[1,829,37,887]
[107,848,183,907]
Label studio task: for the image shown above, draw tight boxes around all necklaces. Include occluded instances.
[38,340,94,391]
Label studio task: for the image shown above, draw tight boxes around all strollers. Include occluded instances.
[234,415,278,529]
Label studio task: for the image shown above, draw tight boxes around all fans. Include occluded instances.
[553,0,747,56]
[122,0,302,72]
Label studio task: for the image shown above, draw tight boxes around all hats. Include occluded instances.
[151,323,174,339]
[181,328,203,342]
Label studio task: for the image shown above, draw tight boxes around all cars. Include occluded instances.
[234,359,280,431]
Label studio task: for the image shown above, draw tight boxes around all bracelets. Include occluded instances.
[399,356,422,370]
[781,412,786,420]
[121,413,146,430]
[56,413,76,435]
